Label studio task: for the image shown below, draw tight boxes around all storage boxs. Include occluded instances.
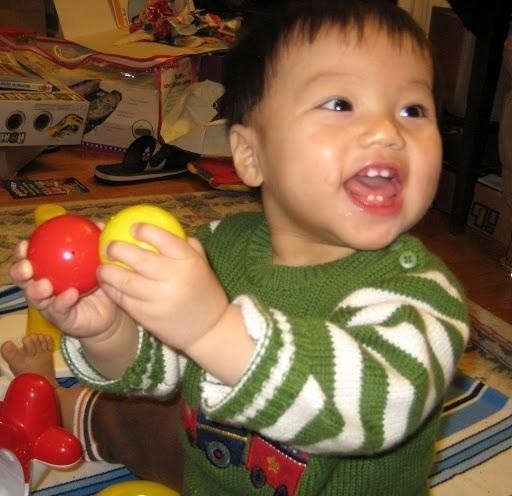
[1,48,90,147]
[467,176,512,248]
[60,58,200,159]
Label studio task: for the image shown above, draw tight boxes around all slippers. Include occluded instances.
[95,136,194,186]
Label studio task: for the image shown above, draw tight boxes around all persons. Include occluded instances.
[0,2,473,495]
